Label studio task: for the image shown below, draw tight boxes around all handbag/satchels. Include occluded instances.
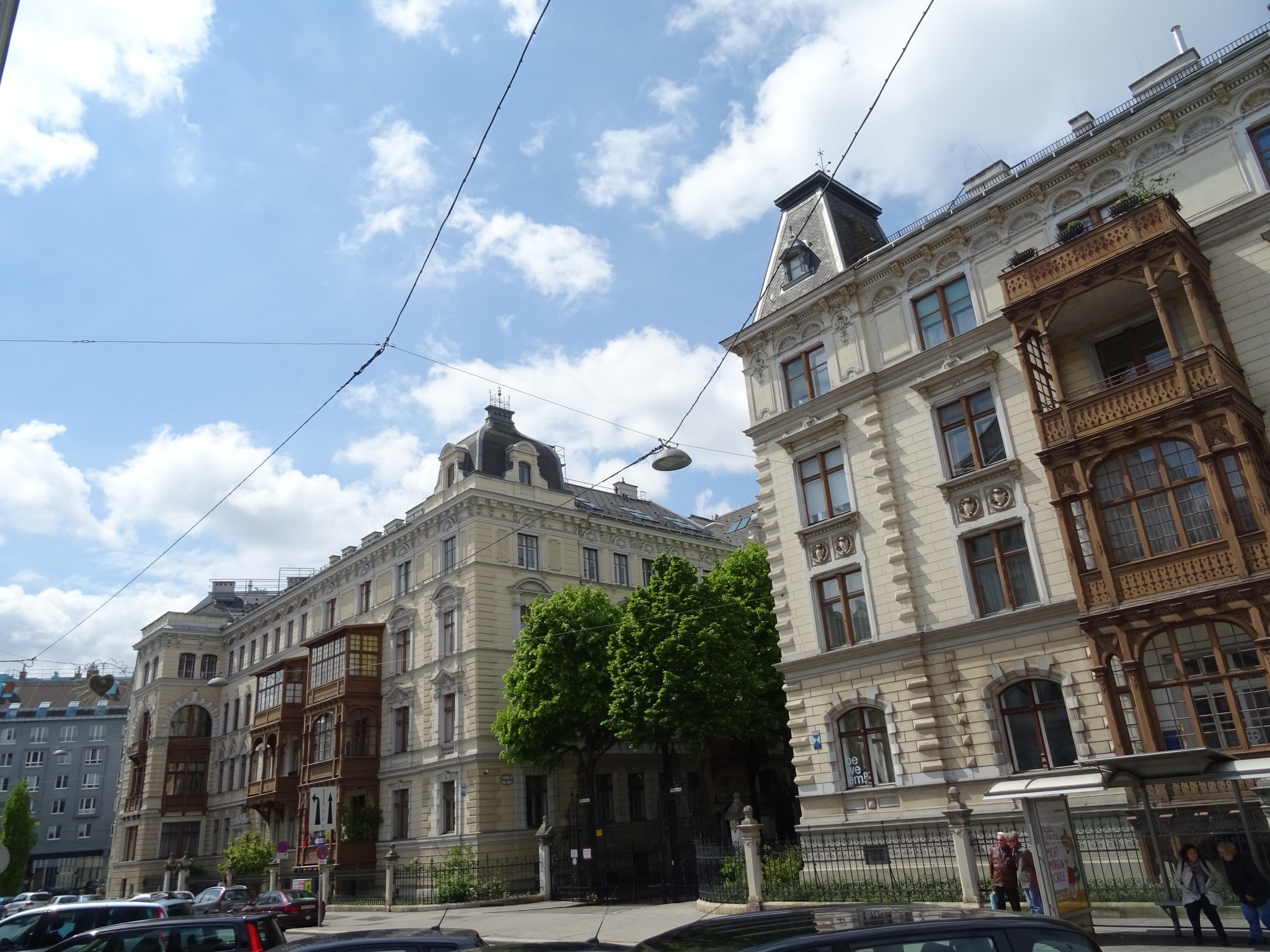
[1239,890,1265,906]
[989,893,998,909]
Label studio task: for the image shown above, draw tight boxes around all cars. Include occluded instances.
[267,905,1104,952]
[0,885,326,952]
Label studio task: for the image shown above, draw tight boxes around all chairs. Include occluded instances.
[44,917,66,947]
[101,933,163,952]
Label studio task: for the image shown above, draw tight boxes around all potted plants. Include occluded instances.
[1165,191,1180,210]
[1057,220,1084,241]
[1110,194,1139,216]
[1010,247,1035,266]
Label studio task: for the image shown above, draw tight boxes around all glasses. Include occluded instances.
[1006,840,1013,843]
[996,838,1003,842]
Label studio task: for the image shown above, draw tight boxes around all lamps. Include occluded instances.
[207,676,229,687]
[652,448,692,470]
[52,749,68,755]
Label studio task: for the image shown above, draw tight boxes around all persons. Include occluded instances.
[17,909,95,949]
[989,831,1021,912]
[1006,830,1044,914]
[218,876,228,887]
[1218,841,1270,940]
[1173,843,1228,941]
[37,888,47,901]
[120,892,125,898]
[95,884,105,897]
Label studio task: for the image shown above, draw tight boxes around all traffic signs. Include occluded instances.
[313,835,325,845]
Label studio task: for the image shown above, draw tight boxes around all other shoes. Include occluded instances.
[1194,936,1204,944]
[1219,933,1230,946]
[1248,938,1257,944]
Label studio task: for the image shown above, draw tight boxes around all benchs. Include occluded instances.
[1154,900,1243,934]
